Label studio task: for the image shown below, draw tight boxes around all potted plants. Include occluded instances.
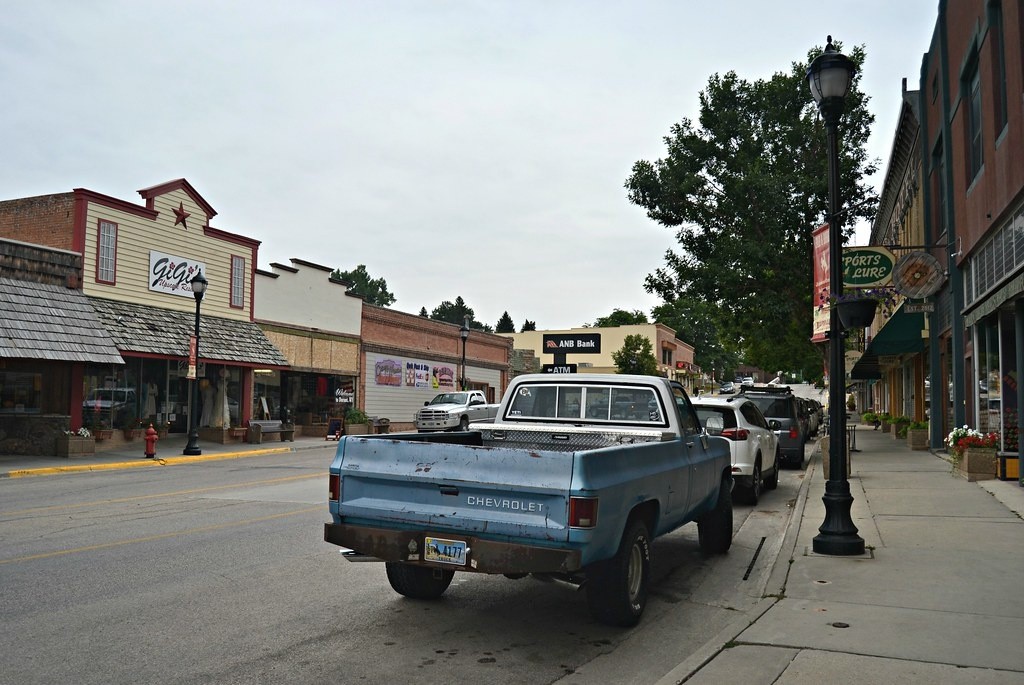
[878,413,891,433]
[886,416,910,440]
[847,402,855,411]
[343,404,370,434]
[898,421,928,451]
[92,416,113,442]
[862,412,877,426]
[153,419,169,440]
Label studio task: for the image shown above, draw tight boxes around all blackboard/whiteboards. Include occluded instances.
[325,418,345,439]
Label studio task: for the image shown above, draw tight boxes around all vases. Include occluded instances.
[57,436,98,458]
[956,447,997,481]
[125,428,145,443]
[833,299,880,330]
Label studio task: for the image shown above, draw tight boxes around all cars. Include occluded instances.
[648,396,658,411]
[227,398,239,418]
[719,382,736,394]
[979,378,987,393]
[567,402,591,412]
[735,376,755,386]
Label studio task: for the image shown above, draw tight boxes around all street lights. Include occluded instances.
[459,324,470,391]
[803,35,867,555]
[183,268,208,456]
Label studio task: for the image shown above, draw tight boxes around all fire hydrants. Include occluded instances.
[144,423,159,458]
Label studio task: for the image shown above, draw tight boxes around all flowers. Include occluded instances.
[818,282,900,320]
[944,425,999,476]
[126,417,147,429]
[63,427,90,438]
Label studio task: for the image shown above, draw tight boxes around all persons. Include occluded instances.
[199,376,230,428]
[145,381,158,415]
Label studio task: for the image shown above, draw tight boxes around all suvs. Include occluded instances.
[737,384,825,470]
[689,394,782,505]
[82,386,136,421]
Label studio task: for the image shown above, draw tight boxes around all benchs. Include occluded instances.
[366,416,390,434]
[246,419,295,444]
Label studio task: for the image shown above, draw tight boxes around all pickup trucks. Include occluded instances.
[589,395,648,419]
[324,373,734,628]
[413,390,501,433]
